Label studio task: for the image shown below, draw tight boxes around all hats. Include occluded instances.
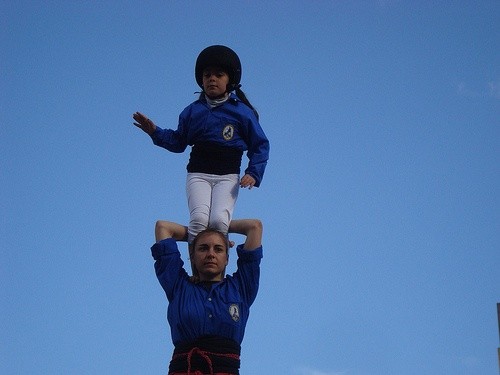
[195,44,241,89]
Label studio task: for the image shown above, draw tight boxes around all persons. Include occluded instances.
[130,45,271,241]
[153,219,263,375]
